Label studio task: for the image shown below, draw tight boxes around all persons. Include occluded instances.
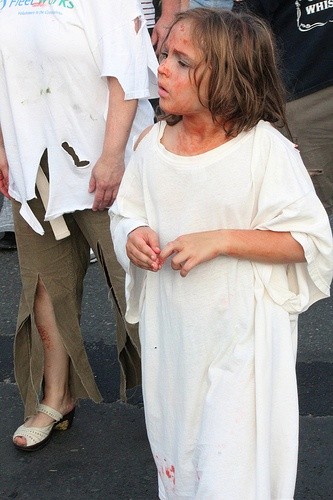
[141,0,235,124]
[0,1,160,450]
[107,7,333,500]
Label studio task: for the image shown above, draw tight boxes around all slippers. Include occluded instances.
[12,404,74,451]
